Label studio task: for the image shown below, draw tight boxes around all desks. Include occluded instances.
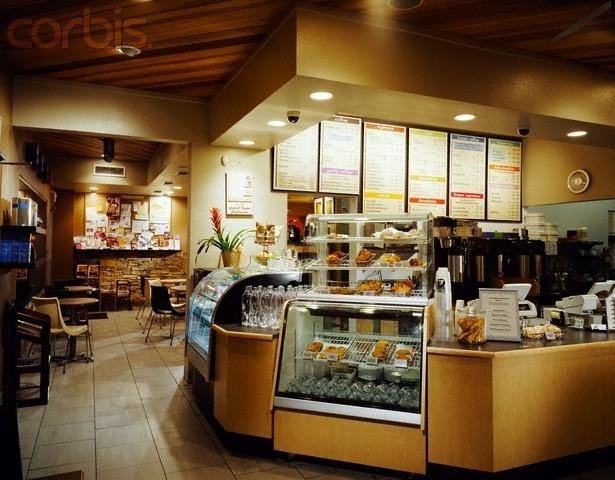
[65,284,93,317]
[57,297,102,361]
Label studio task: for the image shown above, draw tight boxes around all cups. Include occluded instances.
[434,267,452,342]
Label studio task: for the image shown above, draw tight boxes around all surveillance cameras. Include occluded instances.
[287,111,302,122]
[518,128,530,135]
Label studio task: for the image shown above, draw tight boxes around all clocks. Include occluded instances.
[567,170,589,194]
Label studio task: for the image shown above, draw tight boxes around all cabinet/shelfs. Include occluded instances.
[298,212,434,300]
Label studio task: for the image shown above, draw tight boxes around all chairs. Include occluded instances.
[135,271,186,347]
[29,295,93,375]
[115,280,133,311]
[16,306,52,407]
[45,285,74,318]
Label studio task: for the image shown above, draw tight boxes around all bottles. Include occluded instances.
[242,285,309,329]
[456,299,486,344]
[267,248,299,272]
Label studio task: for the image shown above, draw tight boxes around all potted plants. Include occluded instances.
[194,207,257,267]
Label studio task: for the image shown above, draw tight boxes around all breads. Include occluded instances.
[329,281,414,296]
[304,339,411,362]
[324,249,345,267]
[379,254,403,265]
[355,248,371,263]
[457,315,485,343]
